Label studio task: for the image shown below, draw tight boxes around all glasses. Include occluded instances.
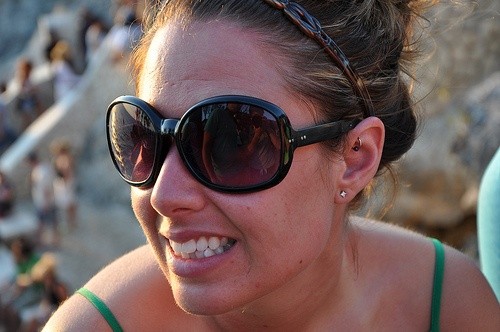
[105,94,363,193]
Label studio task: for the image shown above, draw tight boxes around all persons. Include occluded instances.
[36,1,499,331]
[0,0,168,332]
